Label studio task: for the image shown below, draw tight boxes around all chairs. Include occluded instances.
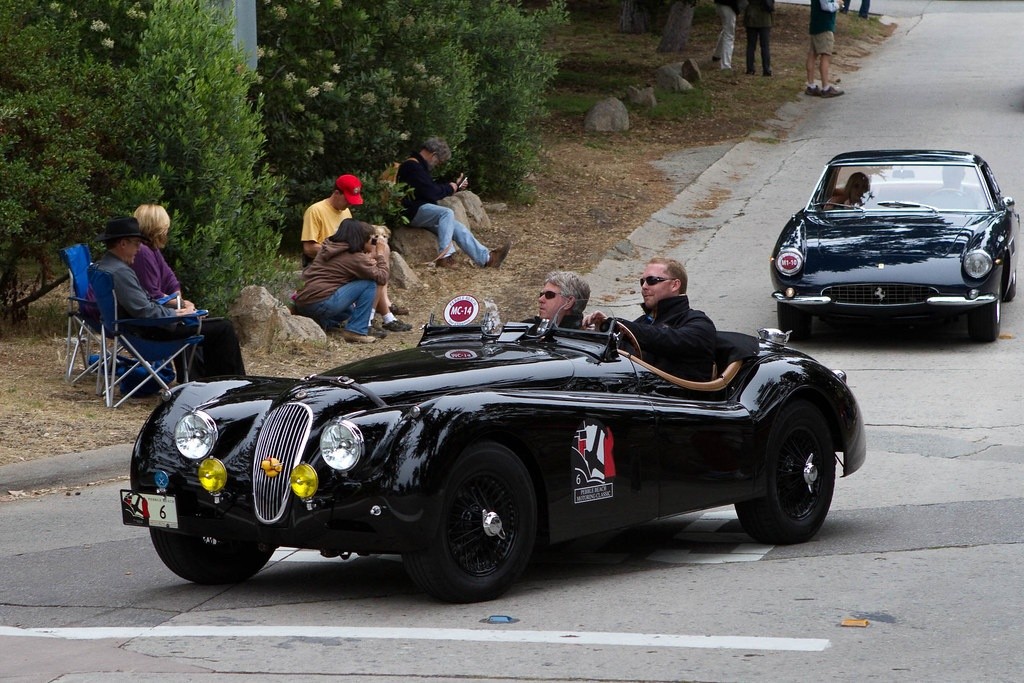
[56,242,207,407]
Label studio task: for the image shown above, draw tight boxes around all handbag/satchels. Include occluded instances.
[118,357,174,396]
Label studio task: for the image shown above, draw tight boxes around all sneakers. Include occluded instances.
[397,319,412,329]
[382,320,407,331]
[436,250,455,267]
[806,85,821,95]
[368,326,386,338]
[821,86,844,97]
[489,241,510,267]
[389,303,409,314]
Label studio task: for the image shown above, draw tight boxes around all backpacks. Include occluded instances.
[379,158,419,208]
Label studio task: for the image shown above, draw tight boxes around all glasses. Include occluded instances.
[640,276,678,285]
[540,291,573,300]
[126,238,142,246]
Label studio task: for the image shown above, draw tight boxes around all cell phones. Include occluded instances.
[455,176,469,188]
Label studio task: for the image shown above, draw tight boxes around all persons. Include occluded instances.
[711,0,742,71]
[135,203,196,311]
[394,137,512,272]
[742,0,778,76]
[805,0,845,98]
[90,215,247,378]
[581,257,715,385]
[927,165,980,208]
[290,174,412,343]
[529,271,591,328]
[822,173,869,210]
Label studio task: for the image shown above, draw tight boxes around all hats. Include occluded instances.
[336,174,363,204]
[94,215,151,242]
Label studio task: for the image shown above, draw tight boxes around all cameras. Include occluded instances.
[370,238,378,245]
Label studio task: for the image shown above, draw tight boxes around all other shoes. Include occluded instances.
[763,71,772,77]
[747,69,755,75]
[344,331,375,342]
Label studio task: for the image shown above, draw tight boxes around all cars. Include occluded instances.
[769,150,1020,341]
[117,295,866,602]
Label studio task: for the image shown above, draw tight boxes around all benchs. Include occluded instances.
[834,180,978,205]
